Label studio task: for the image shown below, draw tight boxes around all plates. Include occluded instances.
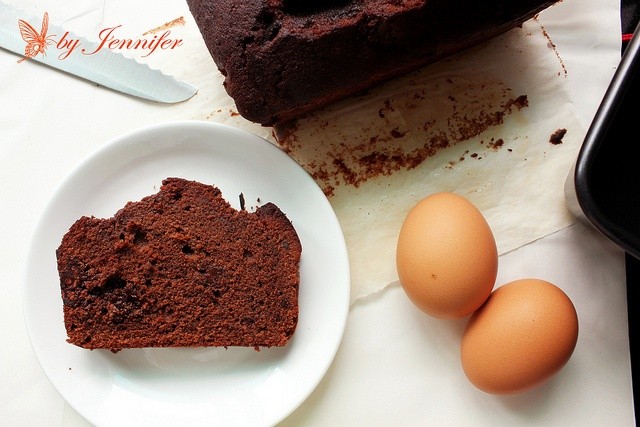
[22,120,351,427]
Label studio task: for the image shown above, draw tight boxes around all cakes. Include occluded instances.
[55,178,301,352]
[185,0,560,126]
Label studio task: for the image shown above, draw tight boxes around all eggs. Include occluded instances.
[460,279,578,396]
[396,193,498,319]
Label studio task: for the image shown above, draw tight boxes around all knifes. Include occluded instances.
[0,0,197,107]
